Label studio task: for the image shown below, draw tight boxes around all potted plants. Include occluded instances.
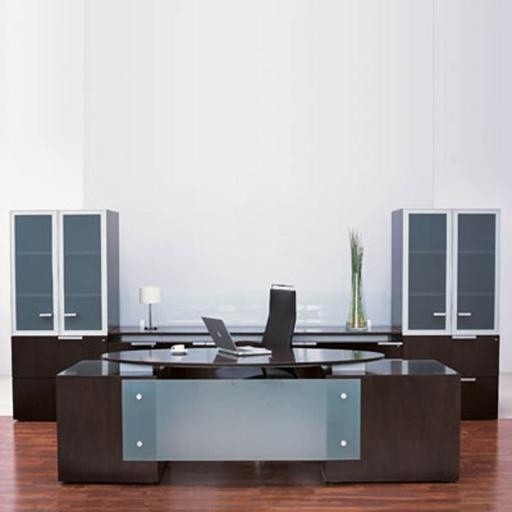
[343,228,369,332]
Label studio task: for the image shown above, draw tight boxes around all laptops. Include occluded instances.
[202,317,272,356]
[212,353,272,367]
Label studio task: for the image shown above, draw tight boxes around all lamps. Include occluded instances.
[139,284,164,329]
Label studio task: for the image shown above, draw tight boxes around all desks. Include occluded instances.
[55,324,461,485]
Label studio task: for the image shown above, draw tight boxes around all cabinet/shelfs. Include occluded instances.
[391,207,500,422]
[9,210,120,423]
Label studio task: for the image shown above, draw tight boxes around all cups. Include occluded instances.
[171,344,184,352]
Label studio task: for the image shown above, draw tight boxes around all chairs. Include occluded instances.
[214,285,297,378]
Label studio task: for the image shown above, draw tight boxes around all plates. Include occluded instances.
[169,350,188,354]
